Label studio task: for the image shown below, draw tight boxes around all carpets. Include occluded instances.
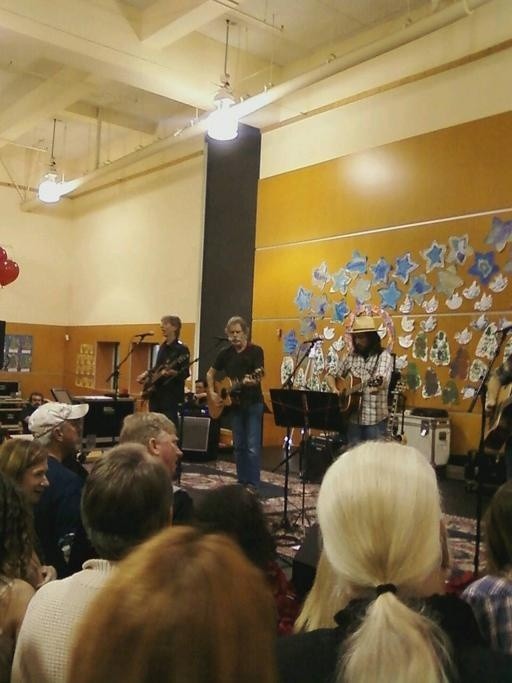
[75,452,500,577]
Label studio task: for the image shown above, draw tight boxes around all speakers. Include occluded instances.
[290,520,323,590]
[180,409,218,458]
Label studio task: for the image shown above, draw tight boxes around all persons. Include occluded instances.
[324,316,393,447]
[193,380,219,443]
[207,316,272,492]
[17,393,43,423]
[137,316,191,435]
[1,401,511,683]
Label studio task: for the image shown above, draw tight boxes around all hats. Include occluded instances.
[27,400,90,440]
[344,316,384,336]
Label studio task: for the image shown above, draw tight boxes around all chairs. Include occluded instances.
[260,547,480,642]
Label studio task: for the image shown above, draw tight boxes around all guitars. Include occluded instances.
[330,372,383,414]
[142,354,189,399]
[204,366,264,421]
[482,382,511,451]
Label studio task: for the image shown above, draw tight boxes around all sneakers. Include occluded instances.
[245,483,257,495]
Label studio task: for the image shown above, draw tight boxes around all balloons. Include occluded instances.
[0,246,19,286]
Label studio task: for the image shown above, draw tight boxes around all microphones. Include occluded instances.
[214,336,232,343]
[134,331,154,336]
[304,337,325,342]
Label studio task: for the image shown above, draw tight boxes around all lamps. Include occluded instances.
[38,117,62,202]
[203,15,239,139]
